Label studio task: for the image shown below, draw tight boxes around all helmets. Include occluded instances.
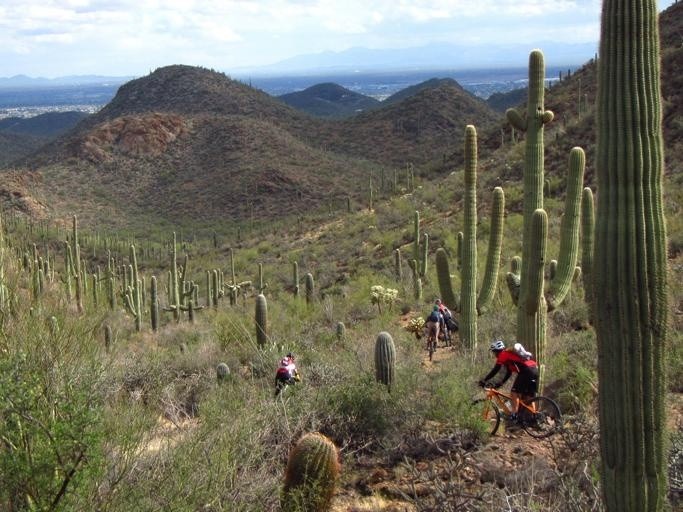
[487,341,506,351]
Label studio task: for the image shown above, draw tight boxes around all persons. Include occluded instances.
[422,298,452,351]
[274,353,301,390]
[476,340,539,428]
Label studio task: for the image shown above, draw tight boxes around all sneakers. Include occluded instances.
[507,412,518,420]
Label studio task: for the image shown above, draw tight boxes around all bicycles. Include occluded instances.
[468,381,562,439]
[443,322,453,347]
[426,326,436,361]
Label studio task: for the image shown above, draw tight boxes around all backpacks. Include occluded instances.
[503,342,532,361]
[280,356,292,366]
[430,311,440,322]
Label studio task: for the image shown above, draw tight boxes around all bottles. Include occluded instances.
[504,399,513,412]
